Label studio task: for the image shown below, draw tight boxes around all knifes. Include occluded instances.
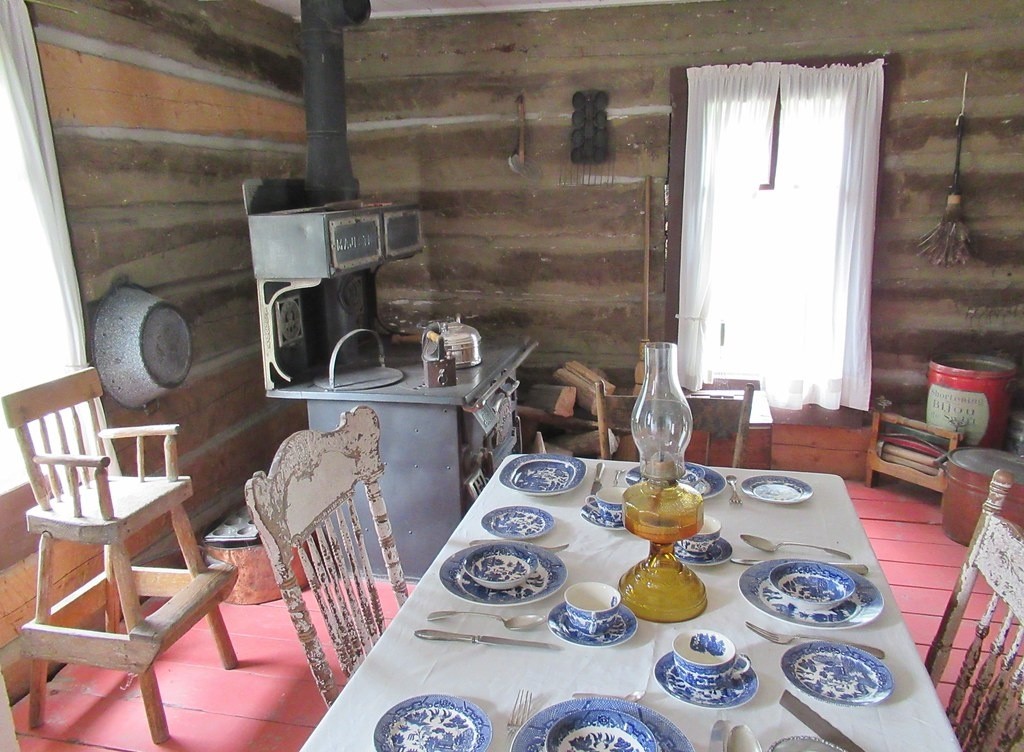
[709,720,723,752]
[589,463,605,502]
[414,630,562,650]
[731,558,869,576]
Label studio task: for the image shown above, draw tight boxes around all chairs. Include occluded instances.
[593,381,755,467]
[920,473,1024,752]
[242,405,406,710]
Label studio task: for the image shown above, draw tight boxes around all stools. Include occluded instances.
[2,369,240,744]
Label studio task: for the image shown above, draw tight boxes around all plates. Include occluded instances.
[547,601,638,648]
[439,453,587,607]
[626,462,814,504]
[581,505,626,530]
[674,537,733,566]
[374,694,696,752]
[654,559,895,710]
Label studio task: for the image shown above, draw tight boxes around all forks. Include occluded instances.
[746,622,885,659]
[469,540,569,552]
[503,690,532,752]
[726,475,742,504]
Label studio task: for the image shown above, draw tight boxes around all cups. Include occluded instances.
[564,582,621,635]
[672,629,751,691]
[677,515,722,554]
[585,486,627,524]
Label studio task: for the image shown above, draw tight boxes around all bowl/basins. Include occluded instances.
[769,561,856,611]
[463,545,539,589]
[545,709,658,752]
[92,275,193,414]
[680,463,705,487]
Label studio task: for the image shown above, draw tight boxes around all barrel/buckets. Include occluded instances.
[926,354,1018,450]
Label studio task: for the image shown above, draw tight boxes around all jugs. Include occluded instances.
[417,313,482,369]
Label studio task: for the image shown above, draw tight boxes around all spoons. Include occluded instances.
[740,535,852,560]
[727,725,763,752]
[427,611,546,630]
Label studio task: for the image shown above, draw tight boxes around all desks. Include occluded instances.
[296,451,963,752]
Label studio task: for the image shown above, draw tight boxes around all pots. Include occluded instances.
[934,447,1024,547]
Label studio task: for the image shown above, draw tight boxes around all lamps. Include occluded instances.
[618,340,707,625]
